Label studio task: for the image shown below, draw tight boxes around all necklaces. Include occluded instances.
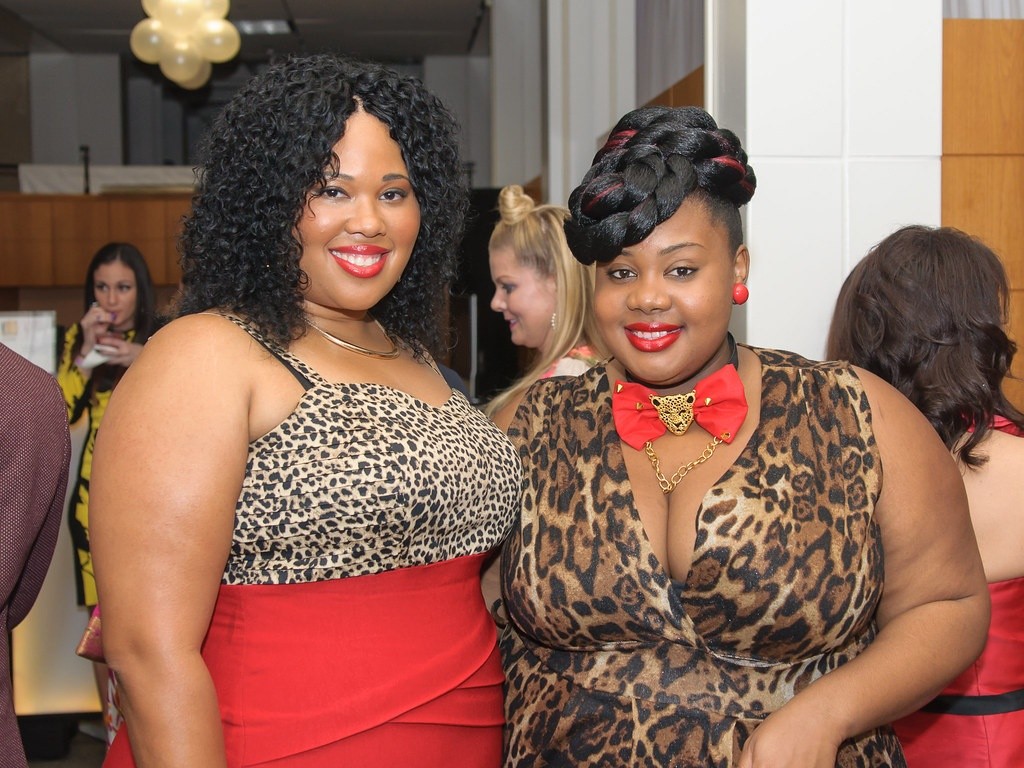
[609,328,750,499]
[302,312,403,362]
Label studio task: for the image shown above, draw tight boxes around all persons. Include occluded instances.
[0,340,72,768]
[817,224,1023,768]
[468,180,605,436]
[49,241,178,733]
[467,99,990,767]
[85,46,521,763]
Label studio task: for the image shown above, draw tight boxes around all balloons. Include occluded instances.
[125,0,241,93]
